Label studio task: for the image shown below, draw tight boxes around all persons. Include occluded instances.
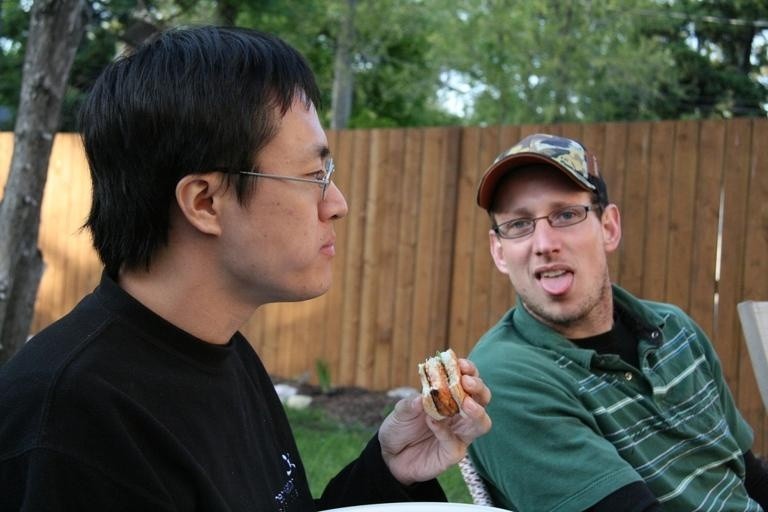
[460,134,768,512]
[0,21,492,512]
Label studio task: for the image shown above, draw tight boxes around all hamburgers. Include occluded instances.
[418,347,470,421]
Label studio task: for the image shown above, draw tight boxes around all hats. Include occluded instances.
[477,134,607,211]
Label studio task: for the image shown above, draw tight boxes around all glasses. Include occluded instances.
[242,157,336,202]
[493,204,599,241]
